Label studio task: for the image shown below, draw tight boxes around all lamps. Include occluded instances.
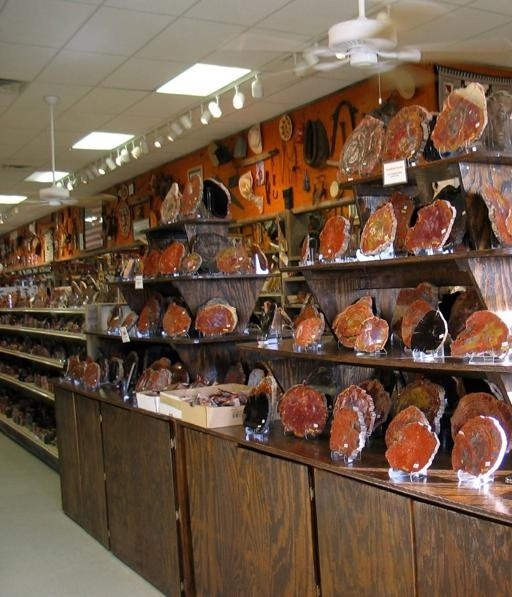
[170,122,183,135]
[154,128,165,148]
[232,84,245,110]
[166,121,176,142]
[199,103,211,125]
[180,109,193,128]
[3,134,149,225]
[251,73,260,98]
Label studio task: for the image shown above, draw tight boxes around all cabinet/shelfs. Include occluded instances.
[0,148,512,597]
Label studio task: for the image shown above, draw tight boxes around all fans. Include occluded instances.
[238,1,464,100]
